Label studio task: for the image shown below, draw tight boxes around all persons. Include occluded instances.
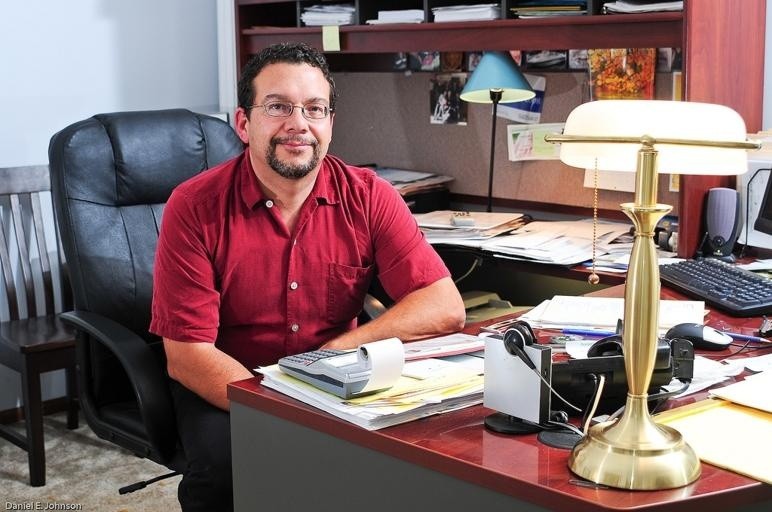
[429,79,468,126]
[393,50,483,74]
[147,40,468,511]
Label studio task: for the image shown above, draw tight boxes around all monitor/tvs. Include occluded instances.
[754,168,772,235]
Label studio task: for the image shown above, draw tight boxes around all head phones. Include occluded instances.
[503,320,537,355]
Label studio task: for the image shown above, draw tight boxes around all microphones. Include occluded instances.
[511,344,583,412]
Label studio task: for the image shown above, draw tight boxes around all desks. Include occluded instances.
[226,199,772,512]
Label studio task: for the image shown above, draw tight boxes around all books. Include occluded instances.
[542,295,705,329]
[301,5,356,26]
[511,7,587,19]
[367,10,424,24]
[432,3,502,23]
[603,1,684,14]
[587,47,657,102]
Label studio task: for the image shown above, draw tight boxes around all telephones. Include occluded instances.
[551,335,673,414]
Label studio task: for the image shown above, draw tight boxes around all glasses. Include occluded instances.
[246,100,332,120]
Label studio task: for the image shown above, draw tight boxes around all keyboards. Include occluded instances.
[659,257,772,318]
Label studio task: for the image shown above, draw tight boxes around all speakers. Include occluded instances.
[703,188,741,263]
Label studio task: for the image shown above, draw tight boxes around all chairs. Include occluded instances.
[0,110,245,494]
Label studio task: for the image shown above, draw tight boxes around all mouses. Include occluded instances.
[665,323,733,351]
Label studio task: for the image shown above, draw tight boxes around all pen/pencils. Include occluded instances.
[723,332,772,343]
[562,329,615,336]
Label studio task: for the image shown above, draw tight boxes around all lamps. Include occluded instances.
[457,50,536,214]
[534,100,761,494]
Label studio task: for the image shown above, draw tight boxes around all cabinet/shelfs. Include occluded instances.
[232,0,766,259]
